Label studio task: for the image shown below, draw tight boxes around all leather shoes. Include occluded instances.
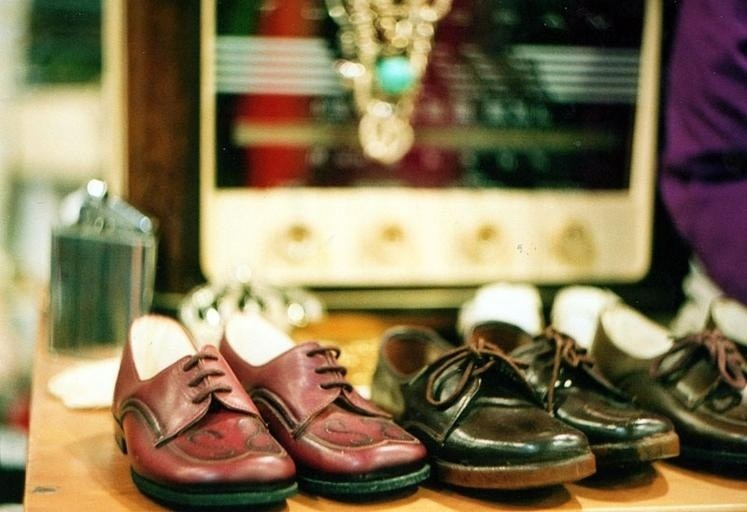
[371,324,595,487]
[113,314,298,503]
[467,320,681,462]
[590,304,744,460]
[219,313,431,493]
